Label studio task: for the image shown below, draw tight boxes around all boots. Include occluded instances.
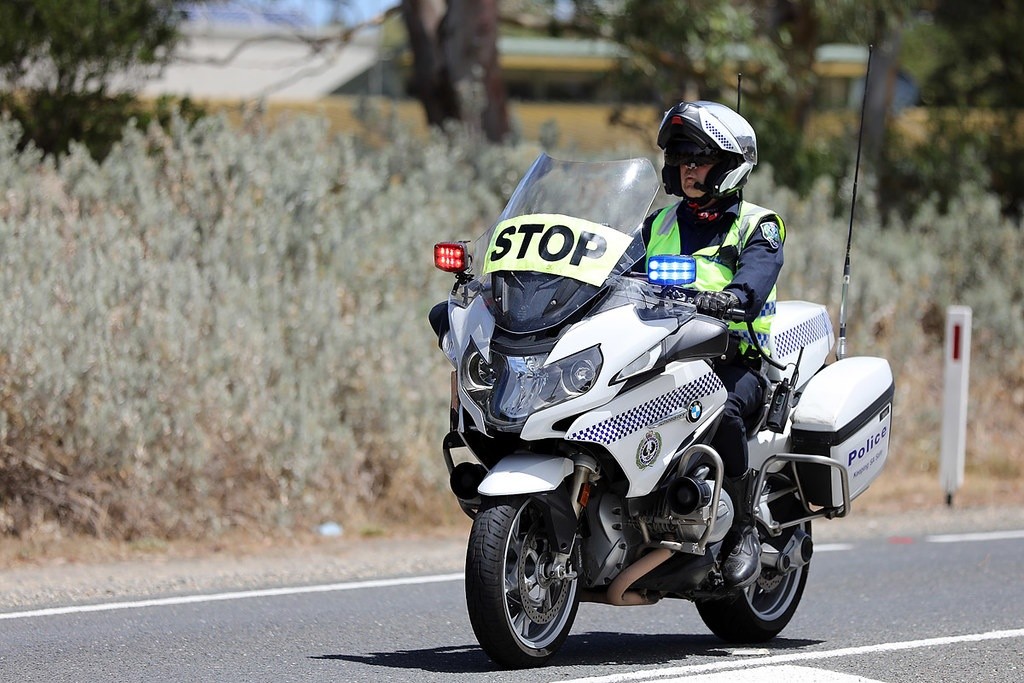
[712,469,773,589]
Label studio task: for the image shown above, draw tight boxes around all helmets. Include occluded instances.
[661,100,758,218]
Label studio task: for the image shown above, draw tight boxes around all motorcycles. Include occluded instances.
[430,149,899,673]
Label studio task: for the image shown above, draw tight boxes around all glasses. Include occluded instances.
[660,139,726,168]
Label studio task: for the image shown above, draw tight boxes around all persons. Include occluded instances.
[622,99,785,584]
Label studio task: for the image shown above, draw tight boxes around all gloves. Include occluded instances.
[692,290,741,324]
